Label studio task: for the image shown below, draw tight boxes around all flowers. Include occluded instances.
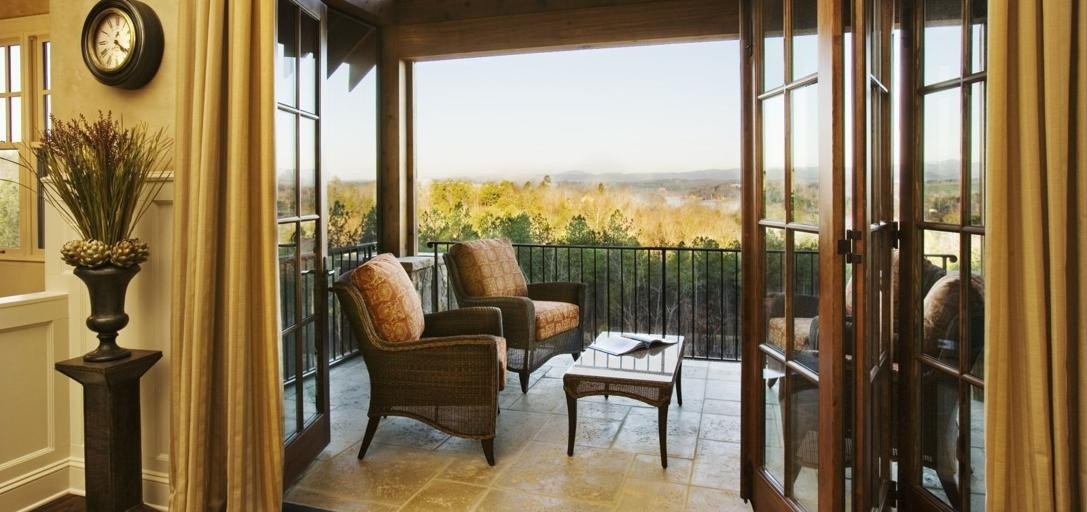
[0,111,176,267]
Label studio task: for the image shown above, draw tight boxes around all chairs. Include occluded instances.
[333,252,507,465]
[766,251,947,388]
[443,239,586,394]
[793,271,985,508]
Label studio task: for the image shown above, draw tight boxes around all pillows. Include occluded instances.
[919,270,982,373]
[347,253,426,345]
[841,256,906,332]
[451,238,528,297]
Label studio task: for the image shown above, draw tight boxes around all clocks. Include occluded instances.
[81,0,165,92]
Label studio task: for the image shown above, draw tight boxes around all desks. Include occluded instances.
[54,349,164,512]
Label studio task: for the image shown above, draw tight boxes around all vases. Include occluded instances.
[73,266,140,363]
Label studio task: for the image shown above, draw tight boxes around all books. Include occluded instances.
[586,331,679,357]
